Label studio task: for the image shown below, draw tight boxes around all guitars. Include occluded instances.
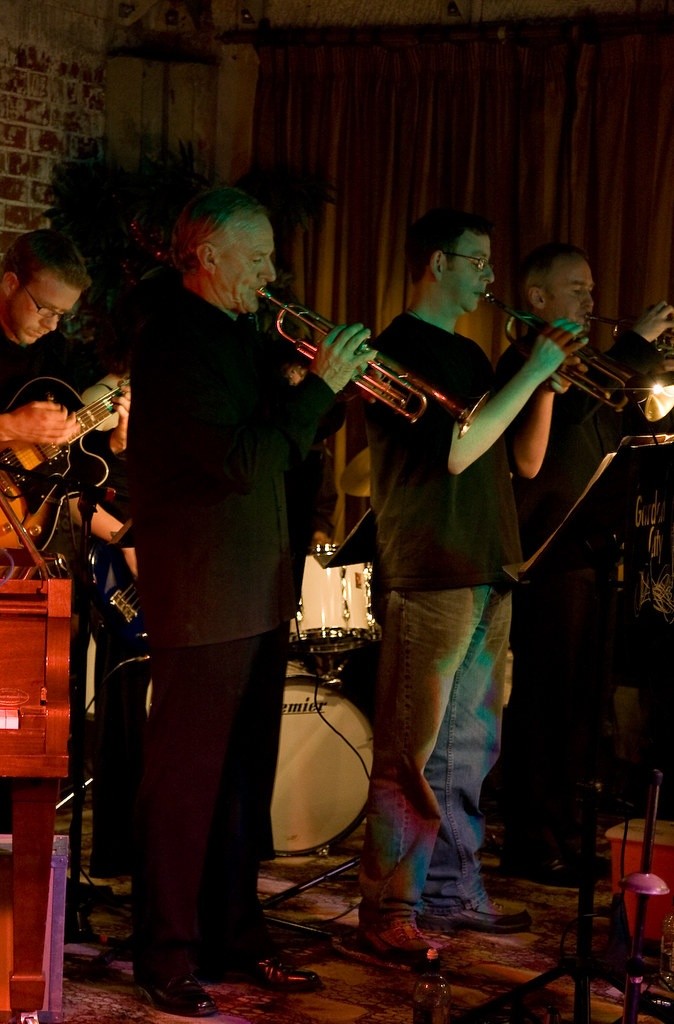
[85,537,154,656]
[1,369,138,549]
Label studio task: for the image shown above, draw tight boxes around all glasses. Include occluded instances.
[19,282,76,324]
[443,249,495,277]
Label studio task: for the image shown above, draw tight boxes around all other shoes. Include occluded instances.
[422,891,533,932]
[500,844,581,889]
[359,915,439,966]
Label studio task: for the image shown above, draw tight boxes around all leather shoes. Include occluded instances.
[198,943,321,992]
[133,965,218,1017]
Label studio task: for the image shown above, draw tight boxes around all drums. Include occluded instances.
[286,540,379,658]
[267,659,378,857]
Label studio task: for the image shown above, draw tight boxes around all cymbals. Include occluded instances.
[339,443,370,497]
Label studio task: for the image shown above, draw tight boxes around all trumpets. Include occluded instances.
[482,289,674,422]
[244,281,486,441]
[583,306,674,359]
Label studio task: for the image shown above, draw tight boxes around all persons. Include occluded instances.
[495,243,674,885]
[357,207,589,973]
[0,229,131,580]
[127,185,377,1016]
